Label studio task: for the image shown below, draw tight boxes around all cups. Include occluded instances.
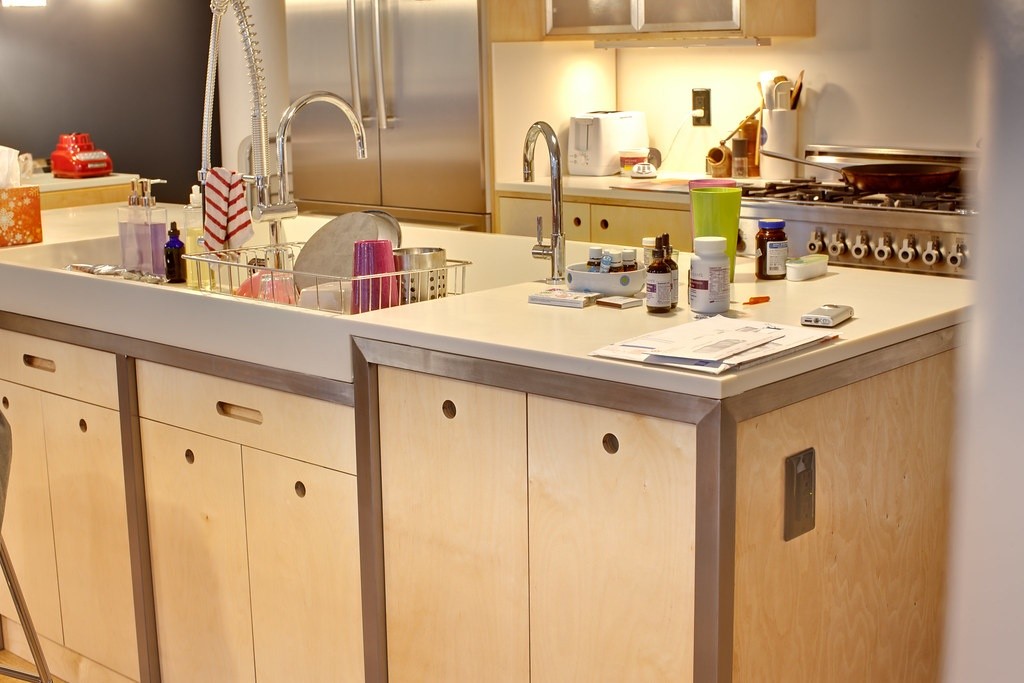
[691,188,742,282]
[256,273,296,306]
[689,179,736,253]
[761,108,797,180]
[618,150,650,177]
[352,240,400,316]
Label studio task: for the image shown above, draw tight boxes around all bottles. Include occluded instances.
[646,233,678,313]
[756,219,788,280]
[164,221,187,282]
[642,237,658,266]
[586,246,637,272]
[733,138,748,179]
[690,236,730,314]
[739,116,760,177]
[183,185,218,293]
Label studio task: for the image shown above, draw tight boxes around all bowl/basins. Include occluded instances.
[292,210,402,296]
[566,262,647,297]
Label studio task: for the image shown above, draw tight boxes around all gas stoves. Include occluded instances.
[737,176,980,279]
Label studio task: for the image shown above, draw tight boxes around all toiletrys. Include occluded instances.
[129,178,167,281]
[163,220,187,284]
[116,179,144,273]
[182,183,218,292]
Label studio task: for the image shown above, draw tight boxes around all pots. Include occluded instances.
[760,150,960,194]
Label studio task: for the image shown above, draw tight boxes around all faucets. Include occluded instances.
[522,120,567,285]
[276,91,368,205]
[197,0,274,206]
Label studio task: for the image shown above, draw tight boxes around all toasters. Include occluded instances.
[567,109,649,176]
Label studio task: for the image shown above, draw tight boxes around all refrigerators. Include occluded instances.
[285,0,495,235]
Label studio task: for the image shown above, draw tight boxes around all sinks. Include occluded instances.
[0,216,653,317]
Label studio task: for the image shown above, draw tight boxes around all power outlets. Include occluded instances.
[785,447,816,543]
[692,87,711,128]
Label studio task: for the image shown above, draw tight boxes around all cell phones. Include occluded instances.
[801,304,855,326]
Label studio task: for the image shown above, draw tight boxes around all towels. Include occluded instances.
[203,167,256,273]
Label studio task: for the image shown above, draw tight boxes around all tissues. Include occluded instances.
[0,146,44,249]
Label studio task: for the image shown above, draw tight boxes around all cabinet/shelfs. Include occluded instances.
[496,191,696,254]
[485,0,817,49]
[0,311,964,683]
